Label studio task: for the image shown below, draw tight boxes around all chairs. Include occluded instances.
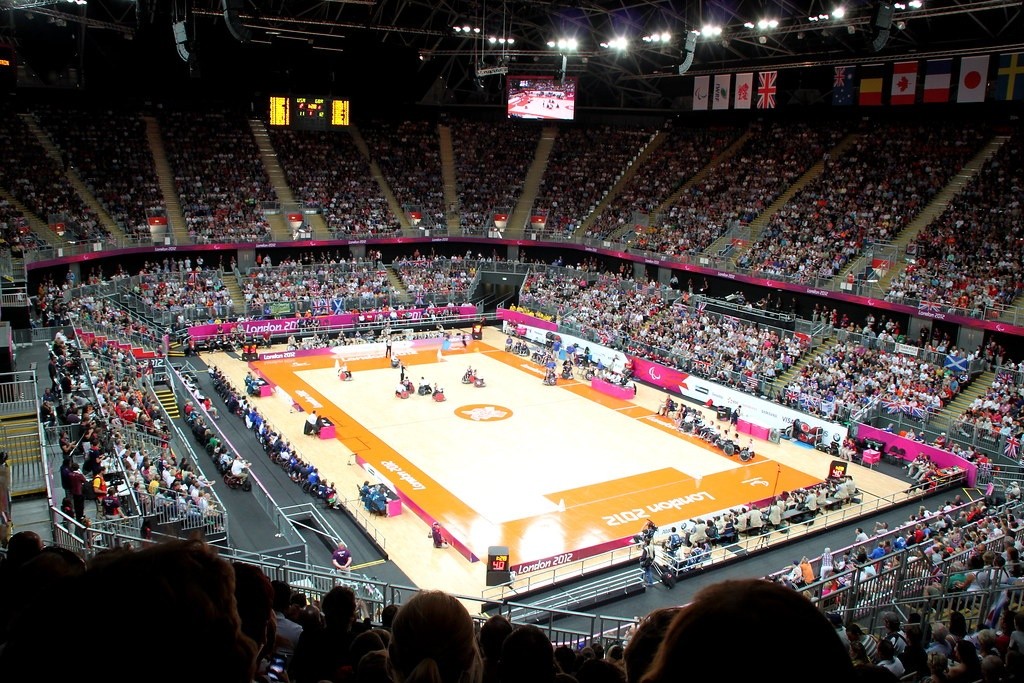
[2,88,1024,683]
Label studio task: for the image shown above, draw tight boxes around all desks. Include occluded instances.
[317,417,336,440]
[368,483,403,518]
[737,416,773,441]
[929,465,965,492]
[254,377,272,397]
[592,376,635,400]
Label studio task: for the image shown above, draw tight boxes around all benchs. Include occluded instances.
[823,490,864,514]
[781,506,821,526]
[738,520,767,539]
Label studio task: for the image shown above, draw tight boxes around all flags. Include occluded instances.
[691,48,1024,111]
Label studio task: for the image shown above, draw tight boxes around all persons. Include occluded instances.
[0,93,1024,683]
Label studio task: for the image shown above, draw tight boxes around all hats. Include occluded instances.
[880,610,900,625]
[826,612,841,625]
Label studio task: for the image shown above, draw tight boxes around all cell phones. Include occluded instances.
[267,652,286,681]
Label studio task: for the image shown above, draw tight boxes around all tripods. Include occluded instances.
[755,536,768,550]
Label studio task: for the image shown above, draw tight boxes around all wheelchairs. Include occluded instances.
[716,438,755,462]
[432,390,445,402]
[461,371,486,388]
[255,425,336,508]
[337,367,353,382]
[505,342,594,386]
[418,383,432,396]
[223,469,251,492]
[395,381,415,399]
